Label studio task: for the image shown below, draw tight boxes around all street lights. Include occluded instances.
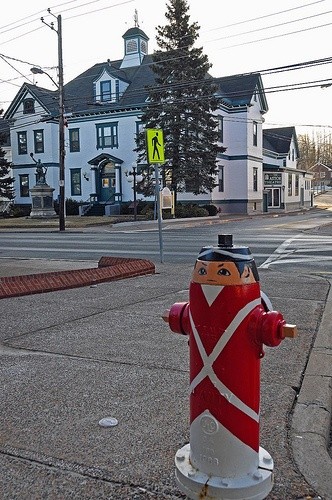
[30,66,66,230]
[123,162,144,221]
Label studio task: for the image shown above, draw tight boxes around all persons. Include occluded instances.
[29,152,47,182]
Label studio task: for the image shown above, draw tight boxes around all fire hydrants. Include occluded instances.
[157,233,300,499]
